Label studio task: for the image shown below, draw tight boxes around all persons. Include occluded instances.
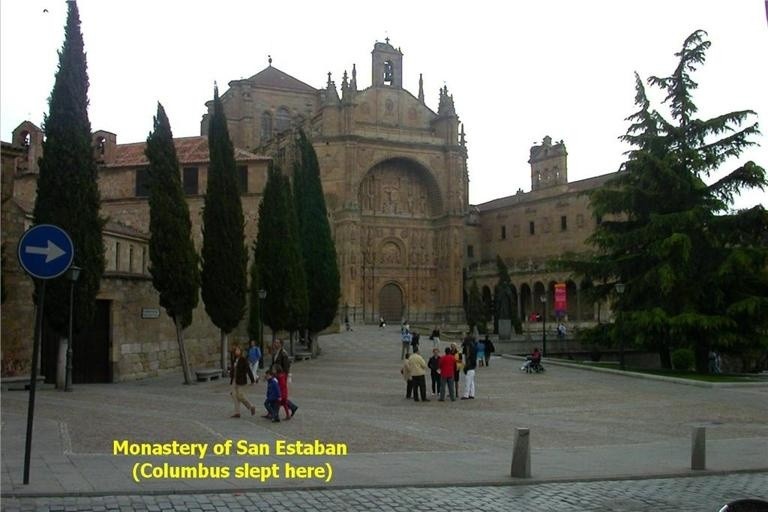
[560,323,566,336]
[710,349,720,376]
[529,312,536,320]
[398,320,496,402]
[536,313,542,322]
[229,337,298,422]
[557,324,561,335]
[519,348,541,371]
[712,350,719,375]
[379,318,386,331]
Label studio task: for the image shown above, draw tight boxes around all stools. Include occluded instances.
[525,363,540,374]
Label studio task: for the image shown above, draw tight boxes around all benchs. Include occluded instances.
[288,356,295,364]
[293,353,312,360]
[195,368,223,382]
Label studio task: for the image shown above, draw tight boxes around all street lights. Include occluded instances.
[540,294,548,357]
[64,262,81,392]
[255,287,268,369]
